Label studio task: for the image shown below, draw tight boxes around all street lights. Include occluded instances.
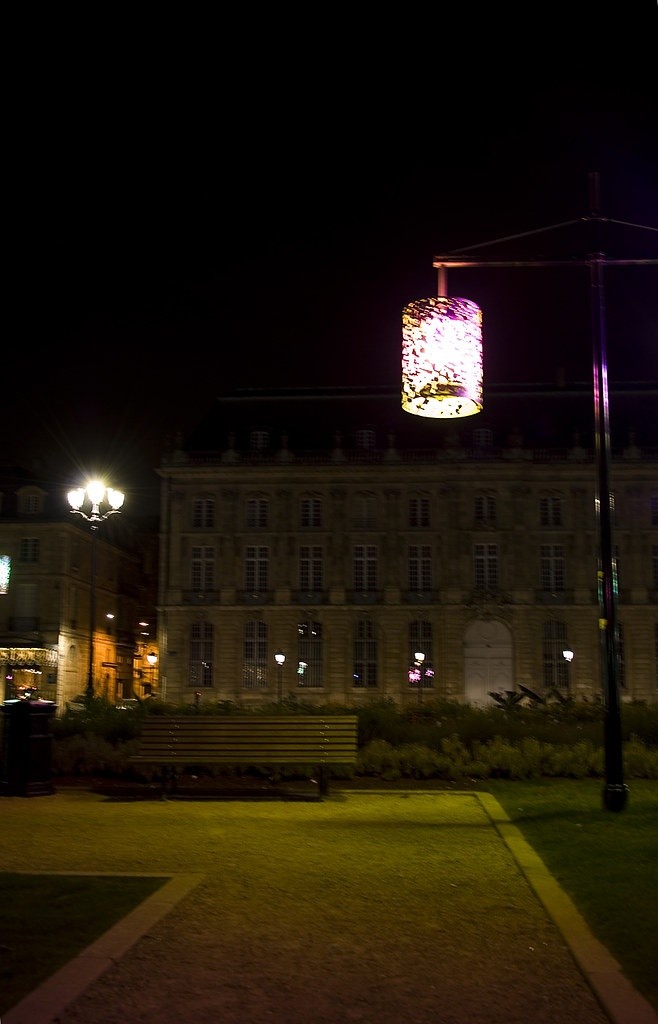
[274,649,286,705]
[399,169,658,815]
[415,649,426,705]
[146,654,158,684]
[67,478,125,703]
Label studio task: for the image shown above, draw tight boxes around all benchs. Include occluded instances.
[125,712,360,797]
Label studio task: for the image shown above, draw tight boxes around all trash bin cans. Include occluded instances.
[1,692,57,795]
